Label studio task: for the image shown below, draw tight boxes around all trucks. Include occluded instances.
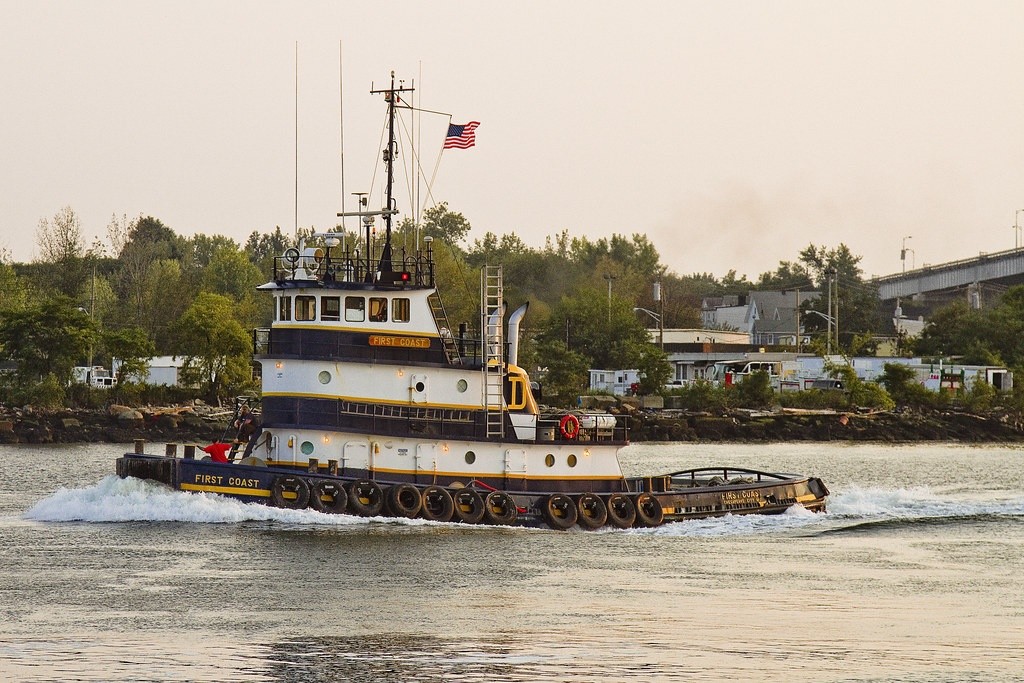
[68,366,116,389]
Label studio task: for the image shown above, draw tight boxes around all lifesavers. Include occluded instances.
[453,488,486,524]
[543,493,579,531]
[635,493,664,527]
[311,479,349,514]
[560,413,580,439]
[270,474,311,510]
[485,490,518,526]
[348,479,384,517]
[420,486,455,522]
[576,493,608,530]
[382,482,423,519]
[607,493,637,529]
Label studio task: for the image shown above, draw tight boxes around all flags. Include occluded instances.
[443,121,480,149]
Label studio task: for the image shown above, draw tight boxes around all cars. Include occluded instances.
[811,379,843,395]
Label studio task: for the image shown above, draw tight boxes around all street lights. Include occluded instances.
[906,249,914,270]
[1013,225,1022,247]
[1016,209,1024,249]
[902,235,912,273]
[633,307,663,355]
[603,274,617,321]
[805,310,839,353]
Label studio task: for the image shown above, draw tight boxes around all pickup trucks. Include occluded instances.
[662,380,687,391]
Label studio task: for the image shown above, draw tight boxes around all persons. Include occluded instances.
[234,406,258,445]
[440,326,453,350]
[196,437,238,465]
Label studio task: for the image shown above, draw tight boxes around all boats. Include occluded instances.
[115,40,830,530]
